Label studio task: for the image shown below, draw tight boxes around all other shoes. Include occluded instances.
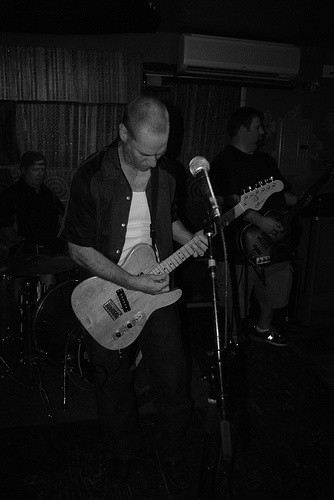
[249,325,288,347]
[227,338,241,355]
[105,457,130,489]
[164,449,192,490]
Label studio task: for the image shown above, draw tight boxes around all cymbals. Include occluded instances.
[1,263,69,276]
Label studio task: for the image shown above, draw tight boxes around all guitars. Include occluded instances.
[69,175,286,353]
[238,194,312,269]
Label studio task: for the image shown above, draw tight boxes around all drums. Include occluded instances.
[32,279,121,390]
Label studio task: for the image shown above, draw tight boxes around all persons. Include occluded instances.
[3,105,314,360]
[59,99,229,482]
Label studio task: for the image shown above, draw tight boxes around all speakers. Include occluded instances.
[284,216,334,330]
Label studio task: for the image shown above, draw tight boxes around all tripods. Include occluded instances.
[0,278,76,423]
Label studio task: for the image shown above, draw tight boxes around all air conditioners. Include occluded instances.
[178,32,301,81]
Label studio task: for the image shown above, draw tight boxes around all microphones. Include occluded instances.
[189,156,223,229]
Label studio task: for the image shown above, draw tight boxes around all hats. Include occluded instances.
[17,151,46,170]
